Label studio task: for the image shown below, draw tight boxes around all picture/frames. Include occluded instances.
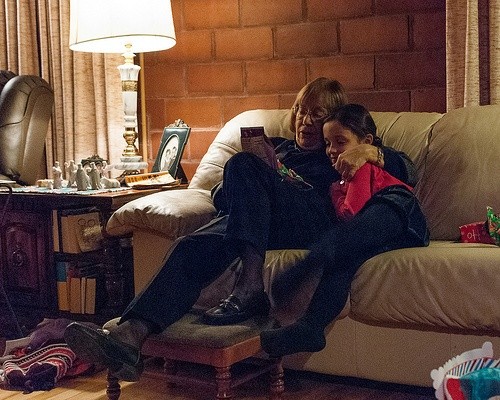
[151,117,189,184]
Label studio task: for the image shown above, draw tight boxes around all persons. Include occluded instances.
[160,147,177,170]
[259,104,430,356]
[64,77,418,382]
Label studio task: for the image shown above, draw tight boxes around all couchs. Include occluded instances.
[108,106,500,392]
[0,70,55,191]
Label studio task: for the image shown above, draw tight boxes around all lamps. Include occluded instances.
[68,0,177,185]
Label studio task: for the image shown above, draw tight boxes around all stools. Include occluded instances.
[102,315,285,398]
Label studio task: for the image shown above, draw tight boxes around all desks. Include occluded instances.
[0,180,190,323]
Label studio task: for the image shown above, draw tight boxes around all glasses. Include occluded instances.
[291,106,330,120]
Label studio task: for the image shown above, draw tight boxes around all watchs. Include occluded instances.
[375,146,382,166]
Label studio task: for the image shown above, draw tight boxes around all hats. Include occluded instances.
[3,338,80,394]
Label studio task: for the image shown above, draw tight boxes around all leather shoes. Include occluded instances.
[202,292,271,324]
[64,322,144,381]
